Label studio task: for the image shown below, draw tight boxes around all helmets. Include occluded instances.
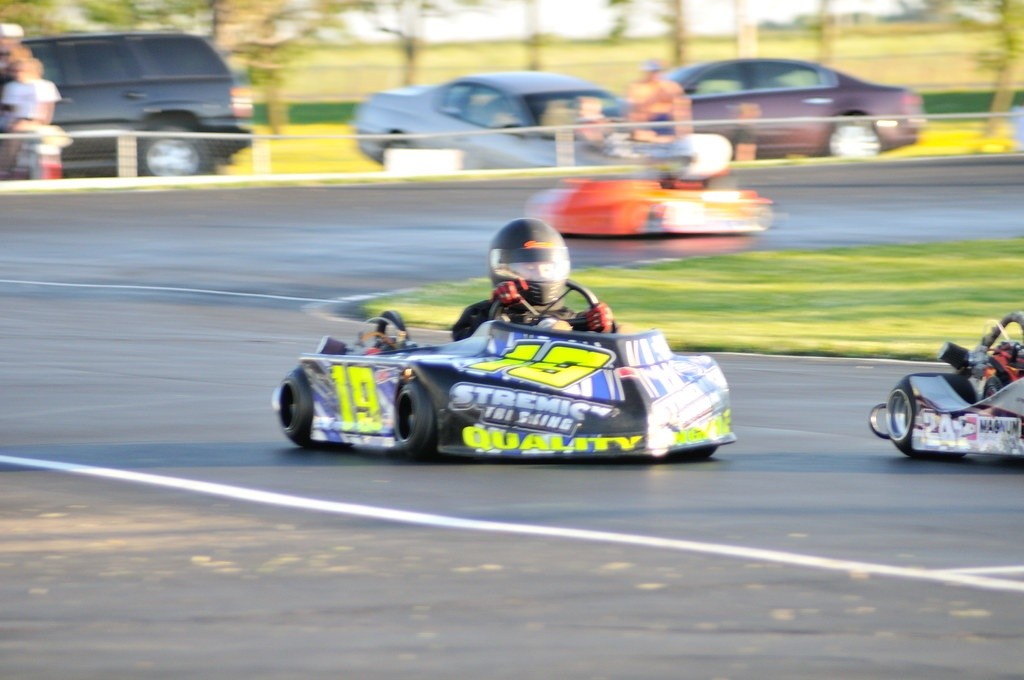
[668,135,733,181]
[488,219,571,306]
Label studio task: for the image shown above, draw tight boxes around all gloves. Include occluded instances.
[491,277,529,313]
[573,302,613,333]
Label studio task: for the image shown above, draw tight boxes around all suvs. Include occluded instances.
[0,32,254,178]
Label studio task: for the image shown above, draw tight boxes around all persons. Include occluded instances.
[0,23,61,178]
[574,97,612,142]
[623,59,692,143]
[663,133,731,190]
[450,218,616,339]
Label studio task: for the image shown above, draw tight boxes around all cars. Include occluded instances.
[625,59,925,162]
[354,72,677,170]
